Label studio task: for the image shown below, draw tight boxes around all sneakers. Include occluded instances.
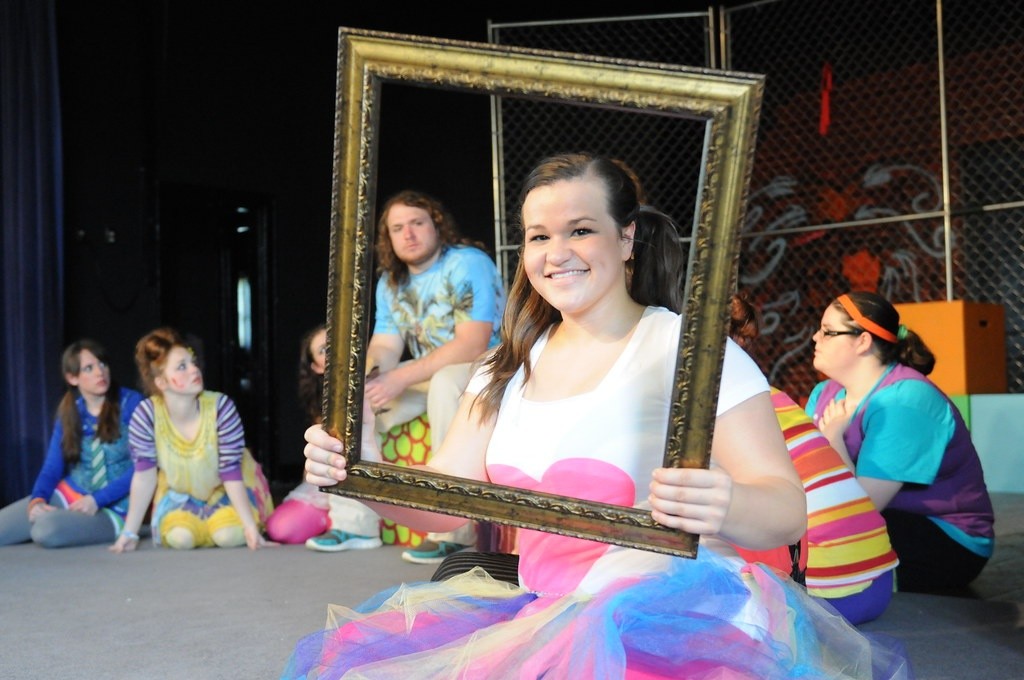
[305,527,383,552]
[401,540,479,565]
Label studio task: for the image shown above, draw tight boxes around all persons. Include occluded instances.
[0,338,145,550]
[281,153,912,678]
[108,327,280,552]
[265,323,333,547]
[803,291,997,596]
[304,190,510,565]
[727,296,900,628]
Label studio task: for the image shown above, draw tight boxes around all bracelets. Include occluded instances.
[119,530,140,539]
[28,496,46,512]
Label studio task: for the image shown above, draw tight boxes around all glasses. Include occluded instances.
[817,322,864,341]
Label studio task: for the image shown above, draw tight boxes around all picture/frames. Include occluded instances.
[319,25,764,559]
